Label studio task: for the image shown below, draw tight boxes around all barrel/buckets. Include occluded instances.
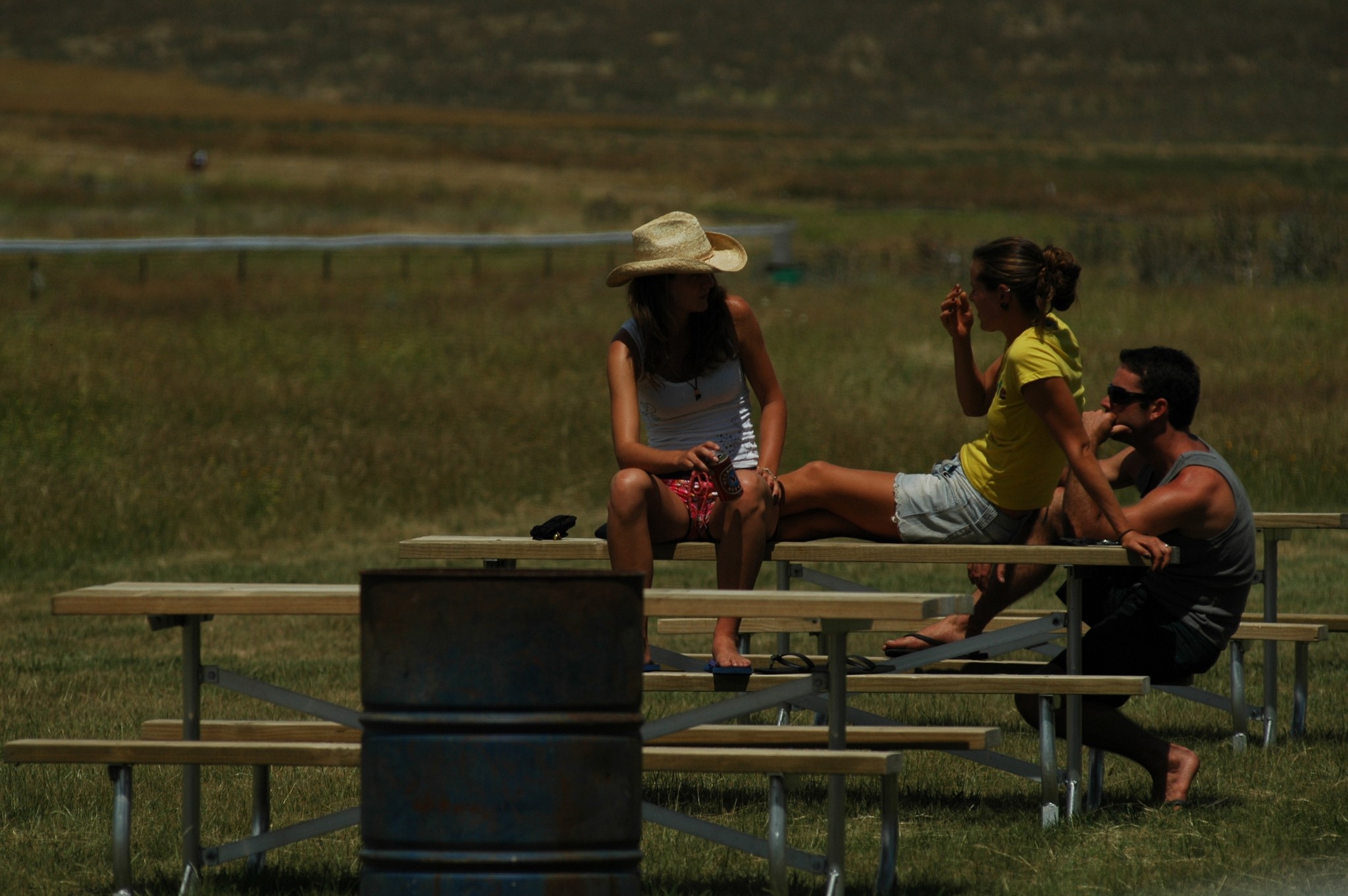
[358,567,651,896]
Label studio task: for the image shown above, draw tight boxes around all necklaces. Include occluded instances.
[663,340,701,401]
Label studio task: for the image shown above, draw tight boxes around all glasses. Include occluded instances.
[1107,384,1156,405]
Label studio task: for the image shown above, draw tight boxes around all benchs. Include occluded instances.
[0,608,1348,895]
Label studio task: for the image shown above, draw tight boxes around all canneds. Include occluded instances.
[706,450,744,504]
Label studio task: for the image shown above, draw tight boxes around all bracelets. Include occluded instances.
[757,466,776,480]
[1118,529,1136,542]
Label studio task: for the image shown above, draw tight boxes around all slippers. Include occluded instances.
[753,653,829,673]
[1160,798,1199,815]
[644,660,661,674]
[810,654,898,674]
[708,659,755,675]
[885,633,989,660]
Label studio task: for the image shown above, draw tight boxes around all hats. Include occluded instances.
[605,212,748,288]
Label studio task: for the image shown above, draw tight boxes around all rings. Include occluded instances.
[1163,544,1168,548]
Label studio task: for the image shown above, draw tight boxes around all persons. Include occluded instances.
[882,346,1256,816]
[773,237,1173,572]
[606,211,788,674]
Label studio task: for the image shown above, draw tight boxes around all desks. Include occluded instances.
[398,535,1177,833]
[51,579,973,896]
[1251,512,1348,750]
